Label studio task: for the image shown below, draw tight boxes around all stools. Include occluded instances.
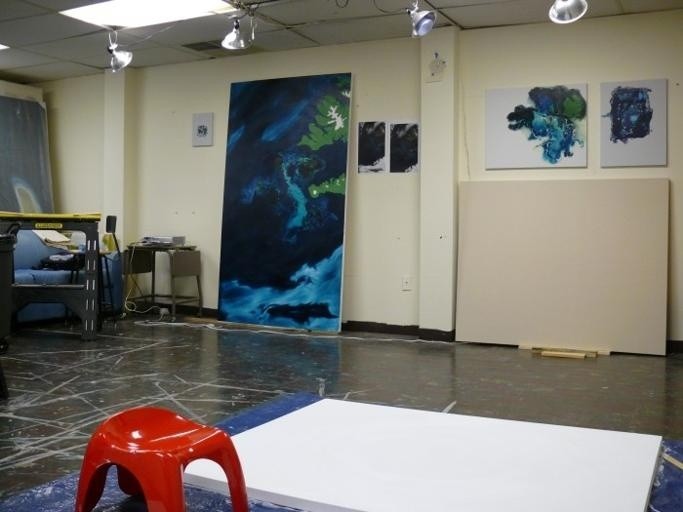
[76,409,251,511]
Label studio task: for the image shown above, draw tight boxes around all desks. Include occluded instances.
[181,397,656,512]
[1,210,102,346]
[120,242,203,325]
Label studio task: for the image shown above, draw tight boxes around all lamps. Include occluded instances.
[105,30,132,74]
[547,0,589,26]
[219,12,254,51]
[403,0,438,40]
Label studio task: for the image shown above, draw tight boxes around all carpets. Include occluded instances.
[0,393,323,512]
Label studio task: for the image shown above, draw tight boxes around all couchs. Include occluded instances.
[11,229,123,322]
